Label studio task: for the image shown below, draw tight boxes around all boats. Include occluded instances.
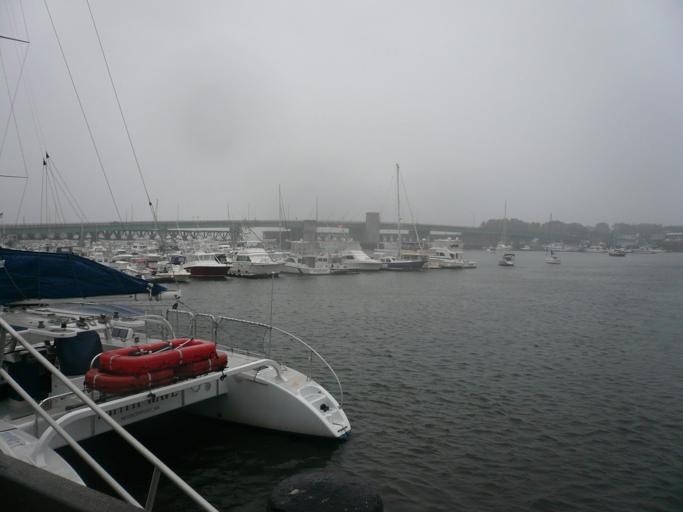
[84,350,228,396]
[606,247,626,257]
[497,251,520,268]
[583,244,607,253]
[1,163,477,288]
[631,246,663,254]
[99,336,216,374]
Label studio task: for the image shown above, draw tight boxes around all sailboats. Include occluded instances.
[541,213,561,264]
[494,199,514,250]
[0,242,349,512]
